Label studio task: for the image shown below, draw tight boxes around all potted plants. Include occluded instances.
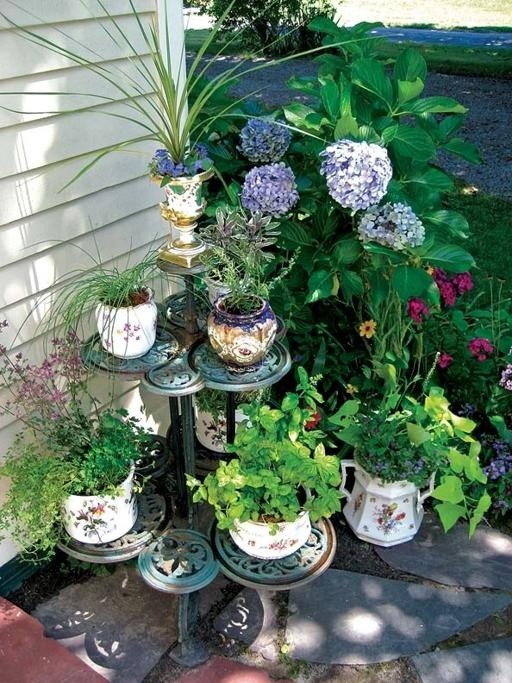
[0,0,492,561]
